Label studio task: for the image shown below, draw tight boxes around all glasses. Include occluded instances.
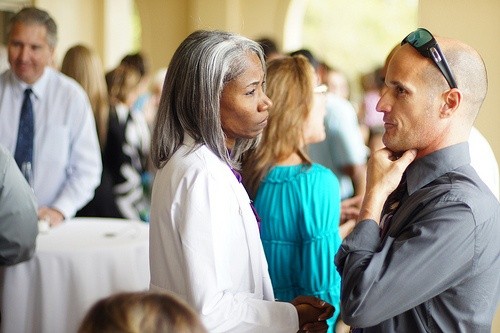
[401,28,458,89]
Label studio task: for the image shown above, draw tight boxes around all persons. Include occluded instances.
[0,145,38,268]
[0,8,102,228]
[239,53,362,333]
[61,46,169,222]
[258,40,366,210]
[334,28,500,333]
[147,30,336,333]
[77,291,209,333]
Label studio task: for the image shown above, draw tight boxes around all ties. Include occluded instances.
[15,88,34,186]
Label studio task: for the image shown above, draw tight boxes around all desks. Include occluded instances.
[0,217,150,333]
